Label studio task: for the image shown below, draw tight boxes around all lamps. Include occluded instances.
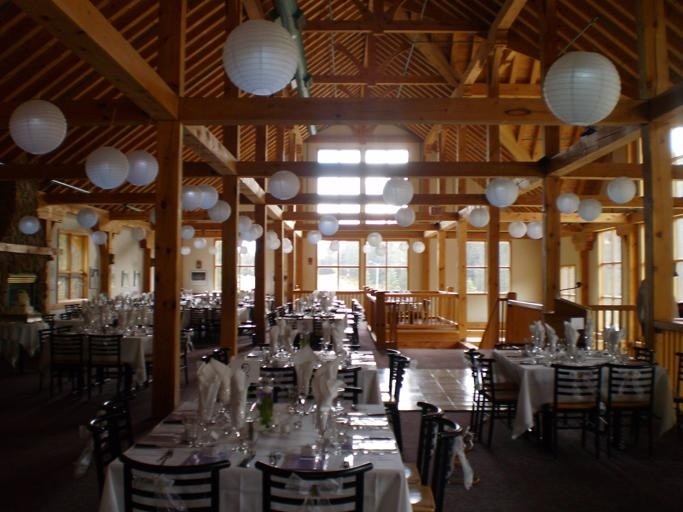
[194,238,206,249]
[517,179,529,190]
[413,241,425,254]
[268,170,300,200]
[469,207,489,227]
[181,246,191,255]
[284,241,292,254]
[78,208,97,228]
[396,208,415,227]
[607,176,637,203]
[526,222,544,240]
[383,177,413,205]
[238,216,263,241]
[182,186,202,210]
[579,199,601,221]
[124,204,144,212]
[208,200,232,222]
[131,227,145,241]
[85,147,129,190]
[0,162,7,166]
[319,216,338,236]
[307,231,321,244]
[18,216,39,234]
[197,184,218,209]
[486,177,519,208]
[182,225,195,240]
[9,100,67,155]
[267,231,280,250]
[125,150,159,187]
[556,193,580,214]
[151,208,157,224]
[544,52,621,127]
[222,19,298,96]
[93,231,107,244]
[368,232,382,246]
[51,179,91,195]
[508,221,527,238]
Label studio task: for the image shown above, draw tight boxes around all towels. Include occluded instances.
[564,322,579,355]
[331,316,347,356]
[311,357,342,437]
[195,361,221,420]
[269,326,278,349]
[229,368,250,428]
[293,346,317,398]
[209,357,232,405]
[607,326,622,354]
[545,324,558,353]
[529,321,544,349]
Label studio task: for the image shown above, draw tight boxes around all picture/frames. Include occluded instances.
[121,271,129,288]
[89,266,99,289]
[134,271,141,287]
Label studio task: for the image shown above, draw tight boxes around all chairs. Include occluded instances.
[306,367,363,404]
[311,316,334,351]
[267,303,293,327]
[119,453,231,512]
[541,363,604,461]
[673,352,683,438]
[60,304,83,320]
[604,363,657,459]
[38,326,59,394]
[87,395,134,502]
[201,348,229,365]
[469,351,520,448]
[189,307,210,345]
[402,401,464,511]
[634,347,654,366]
[179,338,189,384]
[212,308,223,346]
[260,367,297,403]
[42,314,55,328]
[88,334,122,396]
[50,333,83,397]
[343,300,362,351]
[57,325,73,335]
[280,315,304,350]
[380,349,411,452]
[254,461,373,512]
